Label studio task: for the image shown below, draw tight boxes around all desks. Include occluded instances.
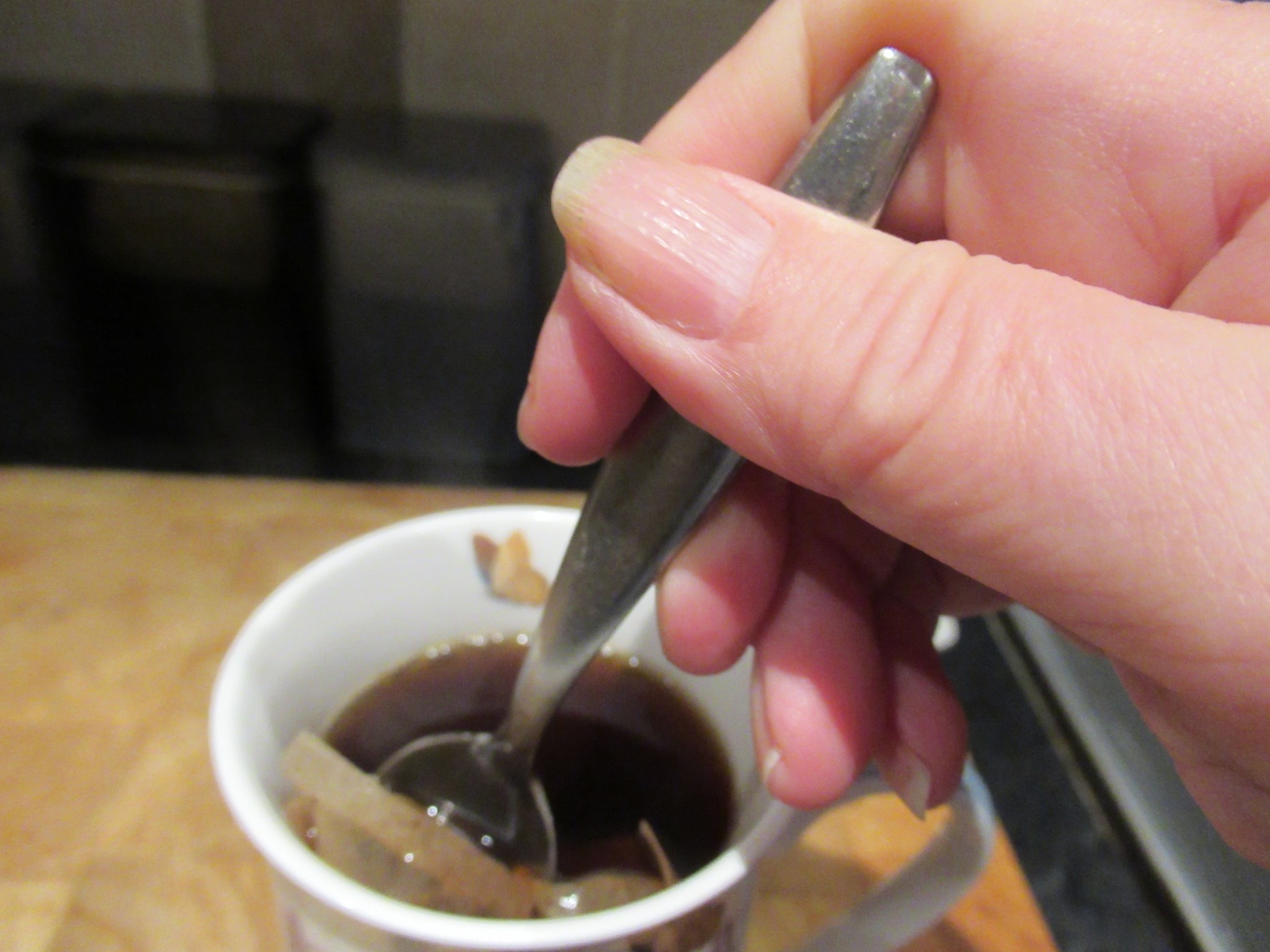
[0,454,1202,952]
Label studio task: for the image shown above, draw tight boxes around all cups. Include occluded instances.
[203,503,996,952]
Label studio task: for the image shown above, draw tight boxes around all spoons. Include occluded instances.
[379,43,939,874]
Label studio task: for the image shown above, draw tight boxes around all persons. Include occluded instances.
[518,1,1269,870]
